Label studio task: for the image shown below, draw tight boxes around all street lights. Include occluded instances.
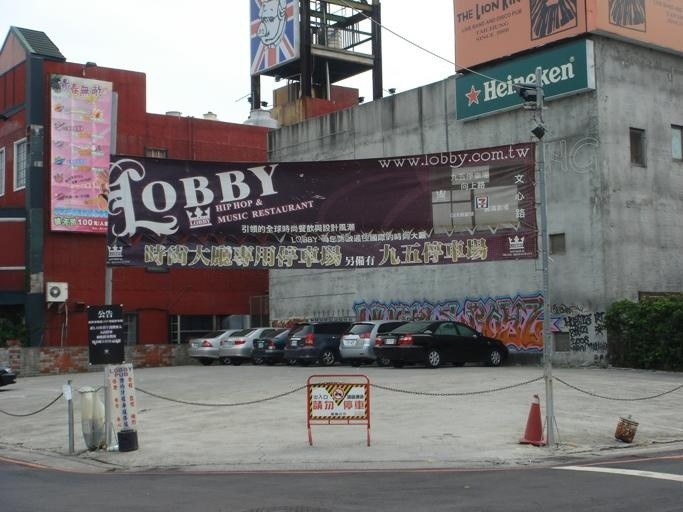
[517,67,563,451]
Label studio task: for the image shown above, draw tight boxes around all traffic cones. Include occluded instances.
[515,393,546,446]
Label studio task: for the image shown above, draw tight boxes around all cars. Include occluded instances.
[0,365,16,388]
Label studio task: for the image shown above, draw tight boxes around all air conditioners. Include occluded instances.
[45,281,68,304]
